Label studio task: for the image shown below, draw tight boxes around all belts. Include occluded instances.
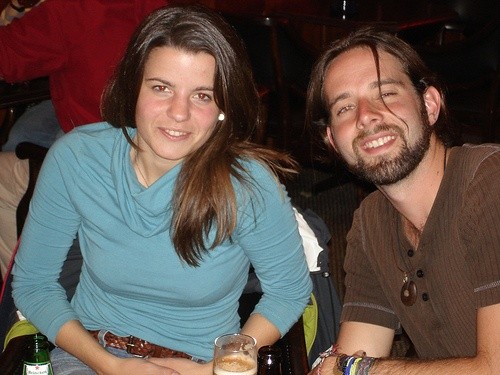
[90,331,206,364]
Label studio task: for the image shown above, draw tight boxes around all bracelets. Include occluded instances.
[315,344,375,374]
[5,2,25,23]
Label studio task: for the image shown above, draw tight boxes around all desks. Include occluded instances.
[261,0,458,185]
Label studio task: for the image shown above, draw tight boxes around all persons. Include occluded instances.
[0,0,182,134]
[304,26,500,374]
[12,10,314,375]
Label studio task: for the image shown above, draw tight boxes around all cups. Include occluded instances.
[213,333,258,375]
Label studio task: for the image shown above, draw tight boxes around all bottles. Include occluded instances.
[20,332,54,375]
[257,345,280,375]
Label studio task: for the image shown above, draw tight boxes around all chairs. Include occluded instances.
[0,140,310,375]
